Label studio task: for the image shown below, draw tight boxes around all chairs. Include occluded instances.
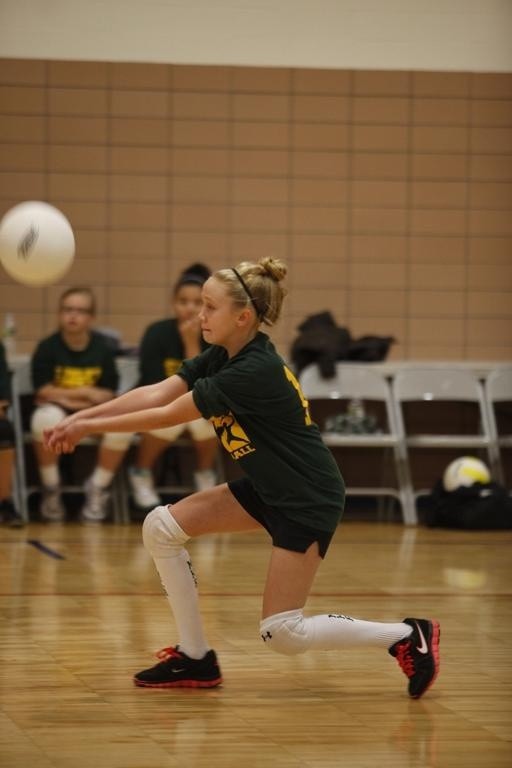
[393,360,494,525]
[10,360,119,527]
[117,356,225,525]
[486,365,512,490]
[299,360,413,527]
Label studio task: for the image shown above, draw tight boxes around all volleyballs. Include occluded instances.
[1,200,77,286]
[443,457,491,490]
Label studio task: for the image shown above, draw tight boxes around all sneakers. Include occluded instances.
[0,498,24,527]
[134,645,225,688]
[194,469,219,493]
[389,618,440,700]
[128,467,160,508]
[80,478,111,522]
[37,489,66,521]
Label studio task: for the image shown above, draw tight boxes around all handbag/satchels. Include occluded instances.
[422,481,512,531]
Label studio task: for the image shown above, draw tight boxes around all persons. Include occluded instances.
[29,286,137,525]
[41,256,442,700]
[126,261,221,513]
[0,342,25,528]
